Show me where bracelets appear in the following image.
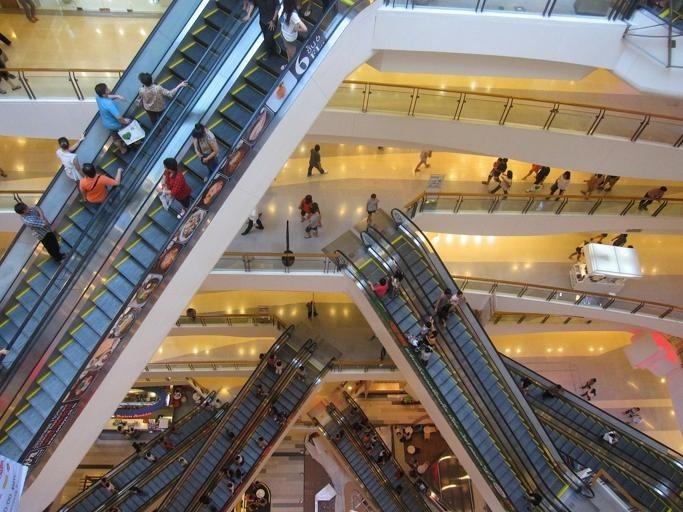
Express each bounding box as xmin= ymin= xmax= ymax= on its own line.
xmin=271 ymin=19 xmax=276 ymax=24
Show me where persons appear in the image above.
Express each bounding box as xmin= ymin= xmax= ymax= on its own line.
xmin=256 ymin=385 xmax=269 ymax=398
xmin=352 ymin=413 xmax=422 ymax=491
xmin=410 ymin=288 xmax=464 ymax=368
xmin=276 ymin=0 xmax=308 ymax=70
xmin=243 ymin=0 xmax=280 ymax=56
xmin=105 ymin=481 xmax=117 ymax=492
xmin=14 ymin=202 xmax=66 ymax=262
xmin=297 ymin=0 xmax=313 ymax=17
xmin=520 ymin=376 xmax=530 ymax=392
xmin=483 ymin=157 xmax=620 ymax=202
xmin=255 ymin=435 xmax=269 ymax=450
xmin=582 ymin=378 xmax=597 ymax=389
xmin=296 ymin=366 xmax=306 ymax=382
xmin=331 ymin=433 xmax=340 ymax=444
xmin=568 ymin=240 xmax=589 ymax=261
xmin=18 ymin=0 xmax=38 ymax=23
xmin=274 ymin=359 xmax=283 ymax=375
xmin=213 ymin=398 xmax=221 ymax=412
xmin=611 ymin=233 xmax=628 ymax=247
xmin=55 ymin=76 xmax=210 ymax=221
xmin=129 ymin=486 xmax=143 ymax=497
xmin=312 ymin=202 xmax=322 ymax=227
xmin=208 ymin=397 xmax=214 ymax=410
xmin=242 ymin=204 xmax=264 ymax=236
xmin=638 ymin=186 xmax=668 ymax=211
xmin=377 ymin=347 xmax=386 ymax=363
xmin=274 ymin=414 xmax=286 ymax=425
xmin=267 ymin=353 xmax=277 ymax=371
xmin=101 ymin=476 xmax=108 ymax=488
xmin=306 ymin=300 xmax=319 ymax=318
xmin=368 ymin=279 xmax=388 ymax=298
xmin=304 ymin=207 xmax=319 ymax=238
xmin=542 ymin=385 xmax=562 ymax=400
xmin=602 ymin=430 xmax=617 ymax=446
xmin=625 ymin=408 xmax=640 ymax=417
xmin=297 ymin=195 xmax=314 ymax=222
xmin=131 ymin=425 xmax=180 ymax=465
xmin=227 ymin=426 xmax=237 ymax=444
xmin=366 ymin=194 xmax=378 ymax=223
xmin=178 ymin=456 xmax=190 ymax=468
xmin=223 ymin=455 xmax=244 ymax=482
xmin=591 ymin=233 xmax=608 ymax=244
xmin=581 ymin=386 xmax=598 ymax=400
xmin=0 ymin=60 xmax=23 ymax=95
xmin=306 ymin=144 xmax=328 ymax=176
xmin=269 ymin=407 xmax=276 ymax=416
xmin=628 ymin=417 xmax=642 ymax=425
xmin=199 ymin=496 xmax=213 ymax=508
xmin=111 ymin=506 xmax=122 ymax=512
xmin=387 ymin=269 xmax=404 ymax=297
xmin=415 ymin=151 xmax=433 ymax=172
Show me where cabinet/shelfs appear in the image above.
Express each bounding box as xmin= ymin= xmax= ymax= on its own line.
xmin=569 ymin=242 xmax=642 ymax=298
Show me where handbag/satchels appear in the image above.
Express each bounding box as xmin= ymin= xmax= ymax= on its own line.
xmin=200 ymin=156 xmax=207 ymax=165
xmin=116 ymin=118 xmax=145 ymax=146
xmin=157 ymin=187 xmax=174 ymax=212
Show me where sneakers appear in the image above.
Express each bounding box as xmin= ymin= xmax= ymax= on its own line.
xmin=579 ymin=182 xmax=615 ymax=197
xmin=306 ymin=169 xmax=329 ymax=178
xmin=300 ymin=216 xmax=323 ymax=239
xmin=637 ymin=206 xmax=649 ymax=212
xmin=240 ymin=224 xmax=265 ymax=236
xmin=481 ymin=176 xmax=564 ymax=202
xmin=176 ymin=208 xmax=186 ymax=220
